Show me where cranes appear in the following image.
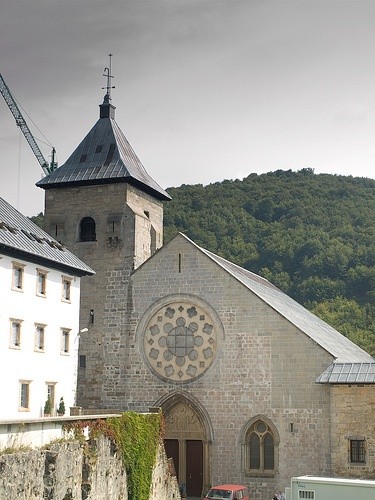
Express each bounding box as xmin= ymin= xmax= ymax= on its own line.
xmin=0 ymin=74 xmax=57 ymax=176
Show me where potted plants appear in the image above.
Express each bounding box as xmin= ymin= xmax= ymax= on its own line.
xmin=56 ymin=397 xmax=65 ymax=417
xmin=44 ymin=396 xmax=52 ymax=417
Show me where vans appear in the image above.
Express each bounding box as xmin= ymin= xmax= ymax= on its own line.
xmin=203 ymin=485 xmax=248 ymax=500
